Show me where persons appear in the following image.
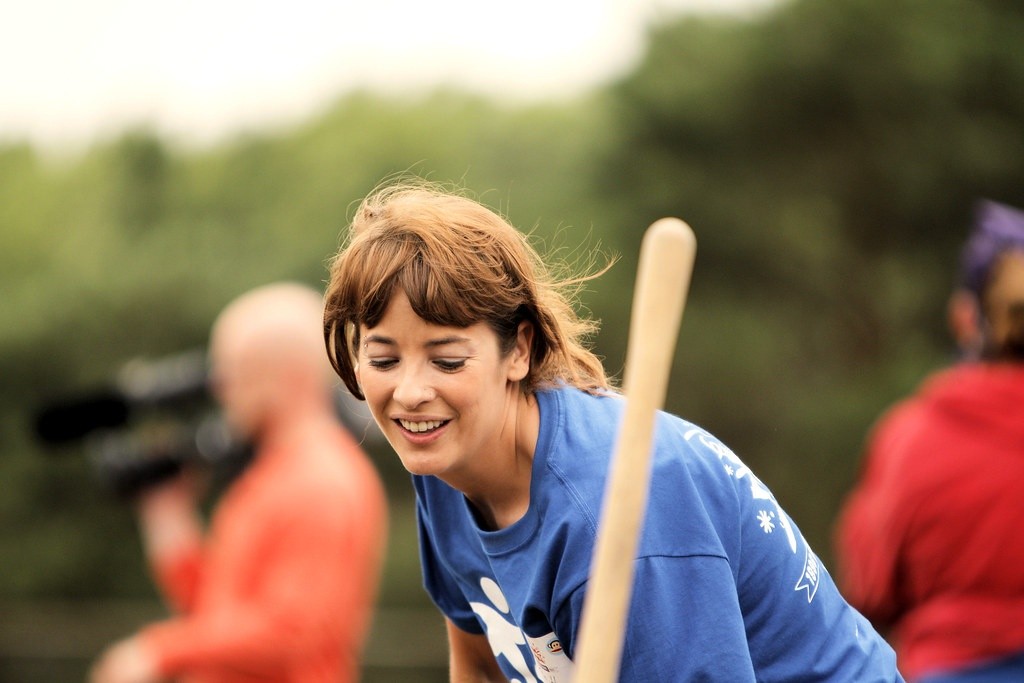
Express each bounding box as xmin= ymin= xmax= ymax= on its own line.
xmin=87 ymin=280 xmax=389 ymax=683
xmin=322 ymin=170 xmax=907 ymax=683
xmin=831 ymin=199 xmax=1024 ymax=683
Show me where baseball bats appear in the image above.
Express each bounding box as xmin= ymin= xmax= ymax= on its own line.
xmin=566 ymin=211 xmax=701 ymax=683
